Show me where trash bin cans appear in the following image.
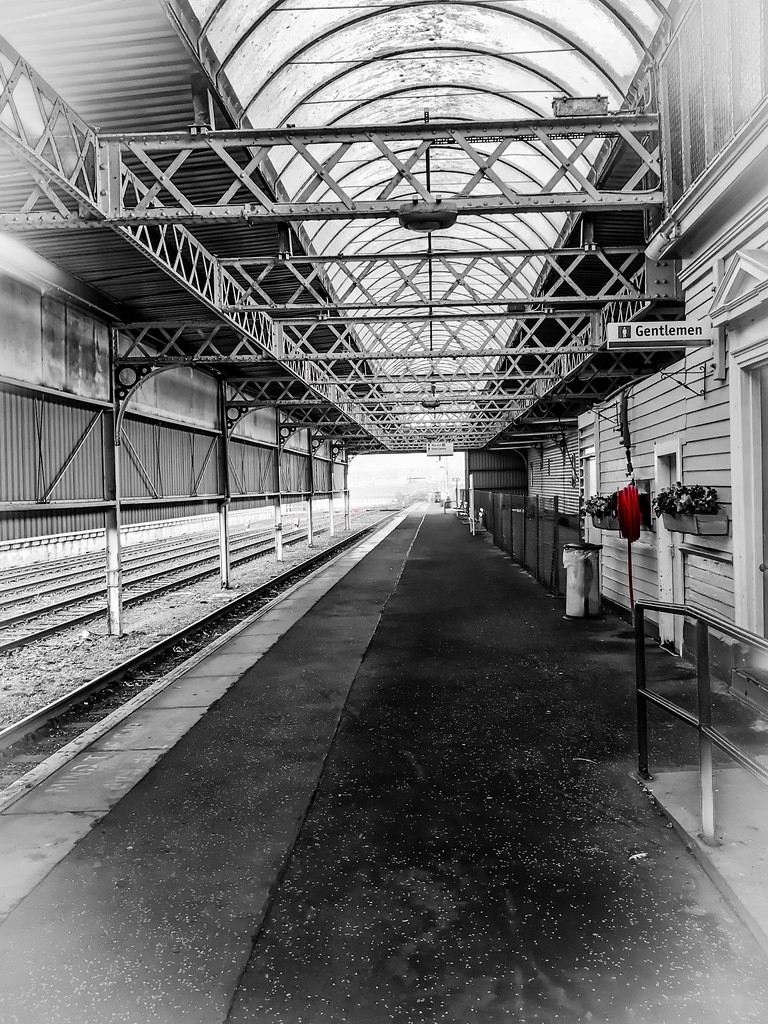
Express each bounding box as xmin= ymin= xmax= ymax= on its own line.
xmin=564 ymin=542 xmax=604 ymax=617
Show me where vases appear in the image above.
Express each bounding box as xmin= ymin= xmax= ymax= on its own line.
xmin=663 ymin=515 xmax=728 ymax=536
xmin=592 ymin=515 xmax=620 ymax=532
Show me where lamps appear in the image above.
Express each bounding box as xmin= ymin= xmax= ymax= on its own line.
xmin=644 ymin=226 xmax=677 ymax=261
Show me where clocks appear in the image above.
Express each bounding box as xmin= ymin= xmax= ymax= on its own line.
xmin=227 ymin=406 xmax=241 ymax=421
xmin=332 ymin=447 xmax=339 ymax=454
xmin=279 ymin=427 xmax=290 ymax=438
xmin=312 ymin=440 xmax=320 ymax=448
xmin=116 ymin=364 xmax=139 ymax=389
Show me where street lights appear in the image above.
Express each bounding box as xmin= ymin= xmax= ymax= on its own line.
xmin=440 ymin=465 xmax=449 ymax=494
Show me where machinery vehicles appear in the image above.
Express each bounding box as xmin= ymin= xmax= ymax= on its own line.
xmin=435 ymin=492 xmax=443 ymax=502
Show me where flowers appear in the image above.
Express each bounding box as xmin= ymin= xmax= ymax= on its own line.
xmin=648 ymin=483 xmax=723 ymax=520
xmin=586 ymin=490 xmax=621 ymax=518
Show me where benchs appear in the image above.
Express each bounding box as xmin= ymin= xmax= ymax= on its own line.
xmin=456 ymin=502 xmax=468 ymax=517
xmin=461 ymin=508 xmax=487 ymax=531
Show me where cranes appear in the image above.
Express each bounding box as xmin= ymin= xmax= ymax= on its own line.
xmin=406 ymin=476 xmax=426 ymax=485
